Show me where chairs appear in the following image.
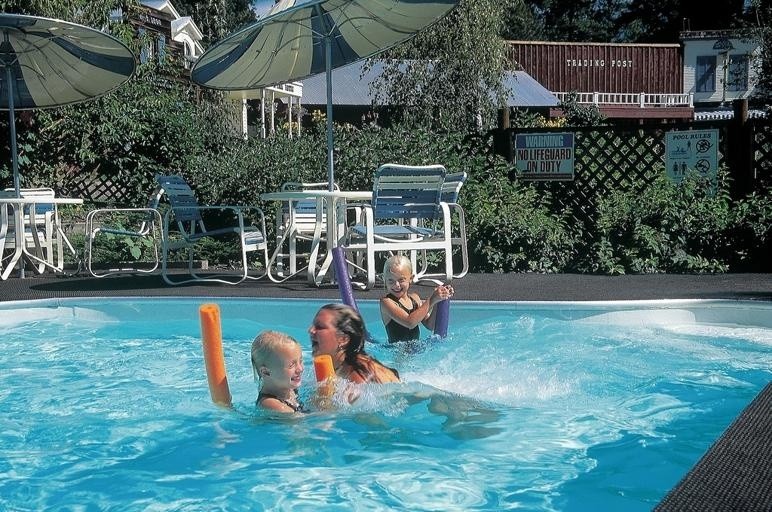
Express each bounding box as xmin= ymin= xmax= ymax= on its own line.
xmin=159 ymin=174 xmax=268 ymax=286
xmin=278 ymin=181 xmax=343 ymax=277
xmin=82 ymin=182 xmax=168 ymax=280
xmin=405 ymin=168 xmax=467 ymax=282
xmin=347 ymin=163 xmax=453 ymax=291
xmin=0 ymin=185 xmax=65 ymax=278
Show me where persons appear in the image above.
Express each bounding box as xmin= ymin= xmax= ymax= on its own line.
xmin=379 ymin=255 xmax=454 ymax=355
xmin=251 ymin=329 xmax=361 ymax=431
xmin=308 ymin=303 xmax=507 ymax=441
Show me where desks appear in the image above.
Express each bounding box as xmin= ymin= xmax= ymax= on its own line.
xmin=262 ymin=187 xmax=403 ymax=287
xmin=0 ymin=197 xmax=88 ymax=281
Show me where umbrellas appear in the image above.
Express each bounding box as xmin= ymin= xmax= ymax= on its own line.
xmin=0 ymin=13 xmax=137 ymax=197
xmin=190 ymin=0 xmax=461 ymax=191
